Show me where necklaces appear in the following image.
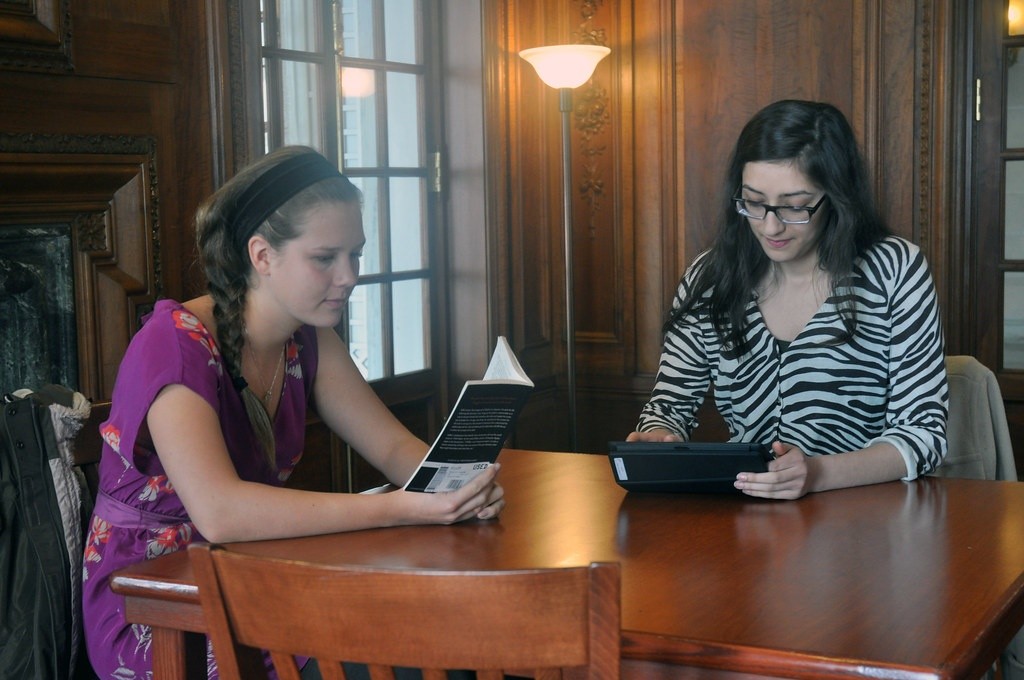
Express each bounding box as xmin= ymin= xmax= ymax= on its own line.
xmin=237 ymin=316 xmax=289 ymax=410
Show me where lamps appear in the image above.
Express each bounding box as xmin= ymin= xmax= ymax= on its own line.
xmin=519 ymin=43 xmax=613 ymax=450
xmin=1008 ymin=0 xmax=1024 ymax=67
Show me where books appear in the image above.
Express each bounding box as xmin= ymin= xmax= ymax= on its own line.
xmin=401 ymin=337 xmax=537 ymax=496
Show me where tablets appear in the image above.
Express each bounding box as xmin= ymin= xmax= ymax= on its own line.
xmin=608 ymin=440 xmax=776 ymax=495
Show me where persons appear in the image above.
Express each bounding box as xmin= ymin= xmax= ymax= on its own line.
xmin=82 ymin=145 xmax=508 ymax=680
xmin=627 ymin=98 xmax=948 ymax=502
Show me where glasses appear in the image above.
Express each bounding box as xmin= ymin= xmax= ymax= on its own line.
xmin=731 ymin=182 xmax=828 ymax=224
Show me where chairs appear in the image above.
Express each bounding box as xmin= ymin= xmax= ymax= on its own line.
xmin=0 ymin=398 xmax=112 ymax=680
xmin=185 ymin=543 xmax=623 ymax=679
xmin=924 ymin=356 xmax=1017 ymax=482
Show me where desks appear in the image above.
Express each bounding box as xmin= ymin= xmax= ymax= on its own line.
xmin=112 ymin=450 xmax=1024 ymax=680
